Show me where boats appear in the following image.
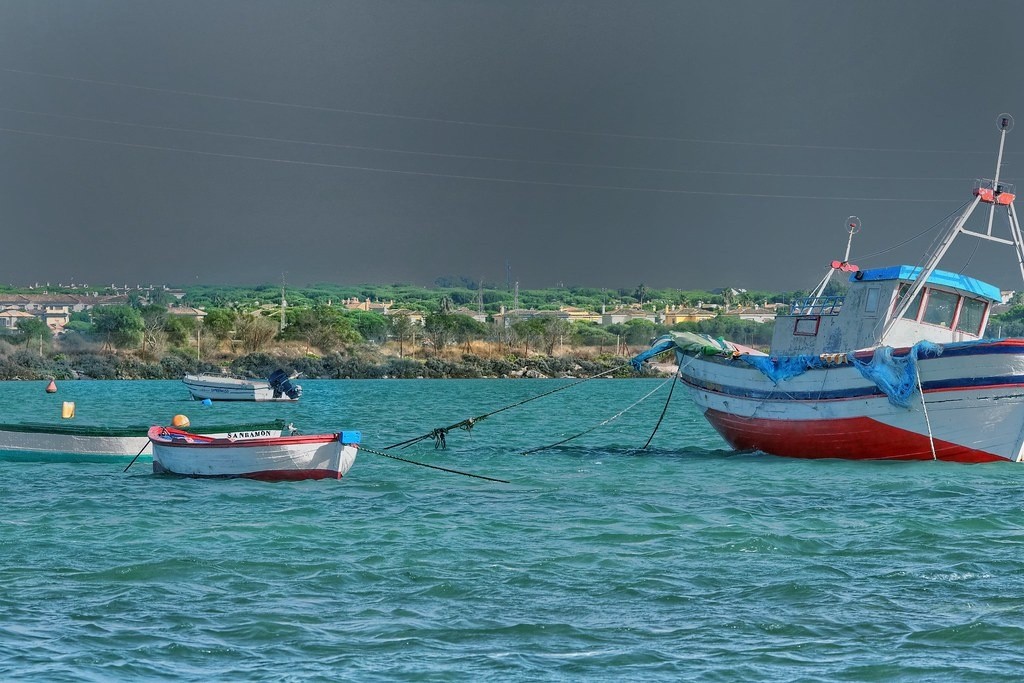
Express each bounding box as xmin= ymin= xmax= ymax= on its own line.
xmin=0 ymin=419 xmax=186 ymax=462
xmin=182 ymin=368 xmax=304 ymax=402
xmin=669 ymin=112 xmax=1024 ymax=462
xmin=148 ymin=424 xmax=360 ymax=483
